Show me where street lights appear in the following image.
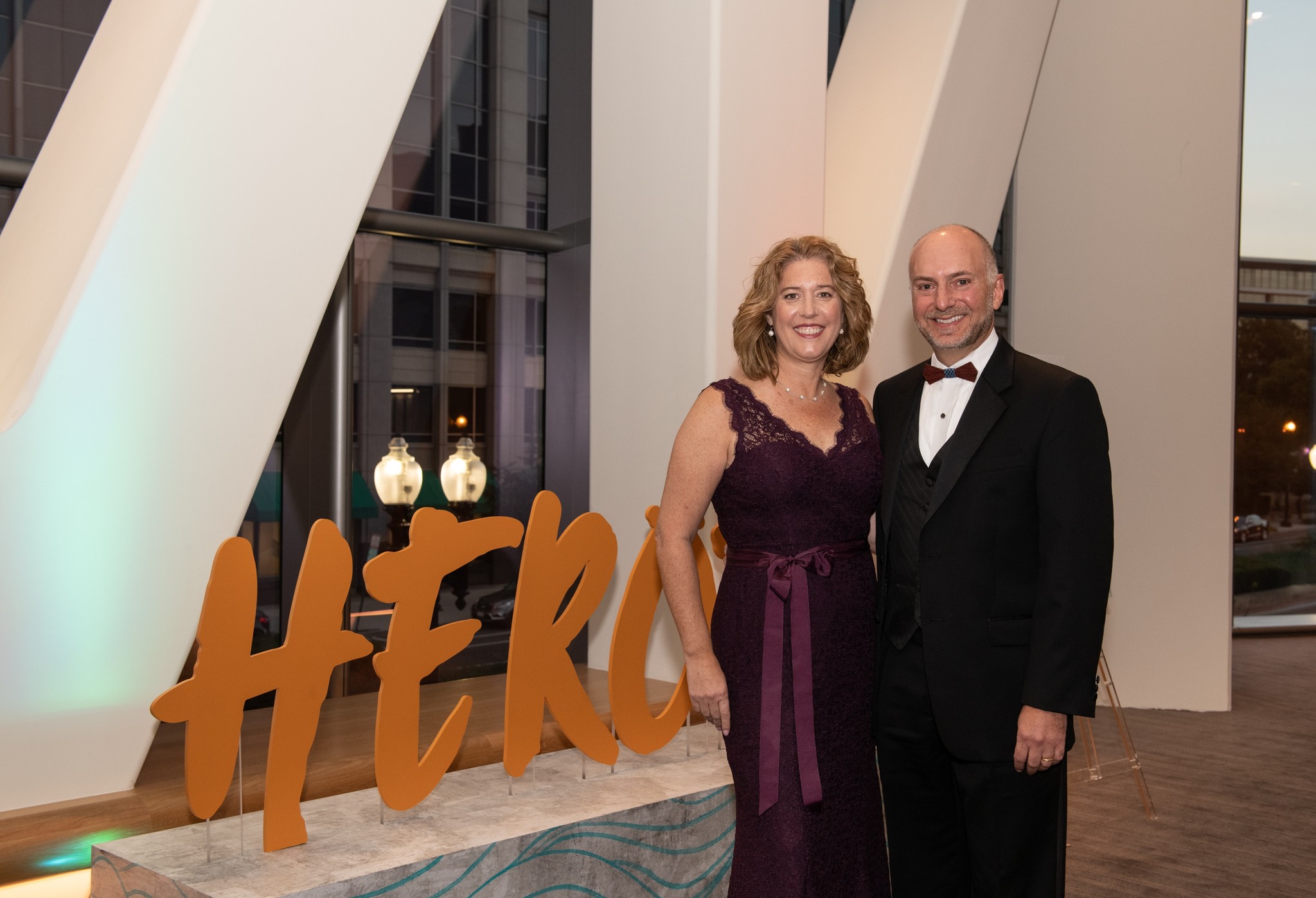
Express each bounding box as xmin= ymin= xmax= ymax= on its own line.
xmin=374 ymin=437 xmax=487 ymax=686
xmin=1280 ymin=420 xmax=1296 ymax=527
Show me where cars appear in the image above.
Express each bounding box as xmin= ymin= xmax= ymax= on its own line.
xmin=1233 ymin=514 xmax=1268 ymax=543
xmin=250 ymin=607 xmax=273 ymax=656
xmin=470 ymin=582 xmax=518 ymax=629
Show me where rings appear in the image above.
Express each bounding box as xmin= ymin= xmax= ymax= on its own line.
xmin=1041 ymin=758 xmax=1054 ymax=761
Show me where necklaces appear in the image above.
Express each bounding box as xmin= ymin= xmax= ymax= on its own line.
xmin=773 ymin=370 xmax=826 ymax=402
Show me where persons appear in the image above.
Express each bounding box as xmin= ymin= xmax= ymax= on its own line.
xmin=656 ymin=236 xmax=876 ymax=898
xmin=872 ymin=222 xmax=1115 ymax=898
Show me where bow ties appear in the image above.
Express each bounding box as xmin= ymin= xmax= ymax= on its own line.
xmin=923 ymin=361 xmax=978 ymax=385
